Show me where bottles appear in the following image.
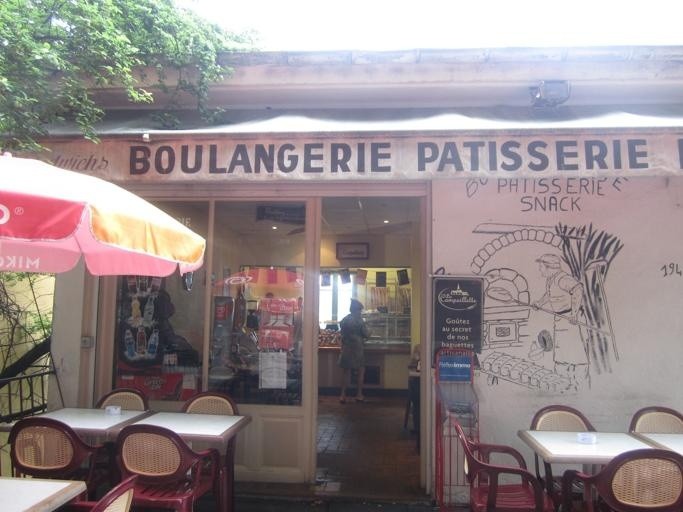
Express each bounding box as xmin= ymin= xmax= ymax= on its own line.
xmin=130 ymin=296 xmax=140 ymax=326
xmin=146 ymin=329 xmax=161 ymax=358
xmin=148 ymin=277 xmax=161 ymax=297
xmin=135 ymin=328 xmax=146 ymax=357
xmin=124 ymin=326 xmax=136 ymax=358
xmin=142 ymin=297 xmax=154 ymax=327
xmin=138 ymin=276 xmax=147 ymax=296
xmin=126 ymin=276 xmax=137 ymax=294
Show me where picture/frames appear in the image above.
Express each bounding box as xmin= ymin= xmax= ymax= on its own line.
xmin=336 ymin=242 xmax=369 ymax=260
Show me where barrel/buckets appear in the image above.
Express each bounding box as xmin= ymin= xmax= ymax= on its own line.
xmin=214 ymin=295 xmax=234 ymax=338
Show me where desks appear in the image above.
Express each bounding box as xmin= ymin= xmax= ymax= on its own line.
xmin=0 ymin=475 xmax=86 ymax=512
xmin=409 ymin=372 xmax=420 ymax=454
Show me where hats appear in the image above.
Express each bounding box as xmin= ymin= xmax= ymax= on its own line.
xmin=349 ymin=299 xmax=363 ymax=312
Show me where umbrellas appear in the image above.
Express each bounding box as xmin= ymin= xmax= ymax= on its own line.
xmin=0 ymin=145 xmax=208 ymax=294
xmin=212 ymin=263 xmax=306 ymax=298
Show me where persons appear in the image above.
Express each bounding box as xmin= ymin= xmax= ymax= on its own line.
xmin=336 ymin=297 xmax=378 ymax=402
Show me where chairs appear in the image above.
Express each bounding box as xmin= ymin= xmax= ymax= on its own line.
xmin=531 ymin=405 xmax=597 ymax=512
xmin=455 ymin=422 xmax=556 ymax=512
xmin=179 ymin=392 xmax=239 ymax=512
xmin=115 ymin=424 xmax=221 ymax=512
xmin=562 ymin=448 xmax=683 ymax=512
xmin=66 ymin=474 xmax=140 ymax=512
xmin=9 ymin=417 xmax=112 ymax=501
xmin=629 ymin=406 xmax=683 ymax=433
xmin=89 ymin=387 xmax=148 ymax=490
